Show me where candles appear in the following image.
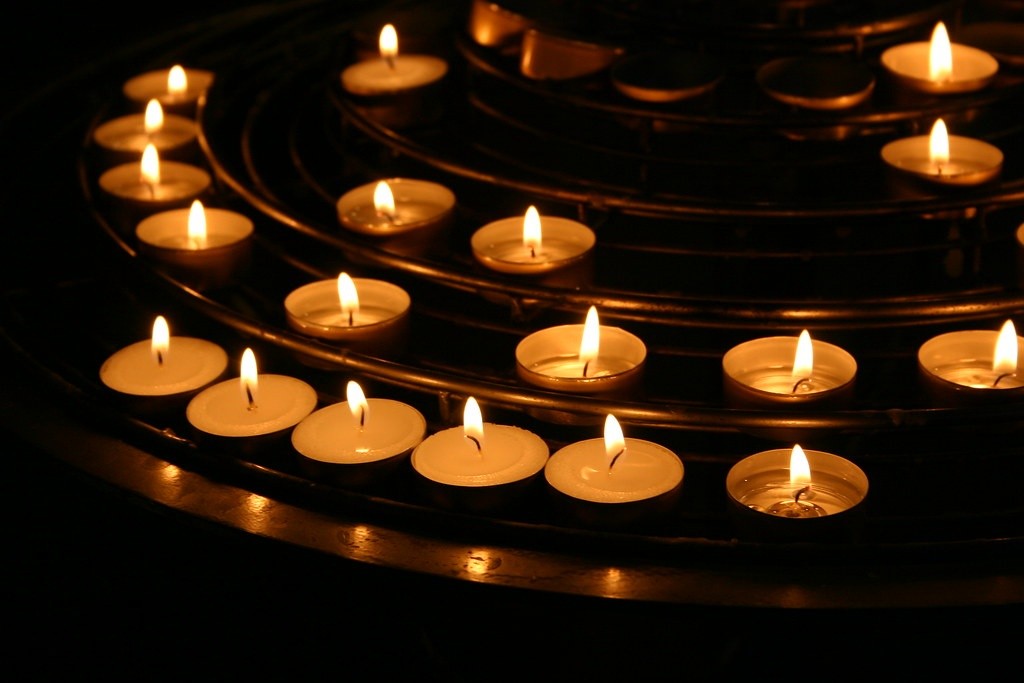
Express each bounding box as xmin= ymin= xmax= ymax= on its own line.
xmin=98 ymin=314 xmax=230 ymax=435
xmin=335 ymin=176 xmax=458 ymax=262
xmin=514 ymin=304 xmax=651 ymax=397
xmin=914 ymin=317 xmax=1024 ymax=411
xmin=879 ymin=21 xmax=999 ymax=109
xmin=407 ymin=397 xmax=553 ymax=516
xmin=721 ymin=327 xmax=861 ymax=412
xmin=97 ymin=143 xmax=213 ymax=245
xmin=90 ymin=98 xmax=201 ymax=183
xmin=542 ymin=414 xmax=687 ymax=536
xmin=468 ymin=204 xmax=595 ymax=292
xmin=338 ymin=21 xmax=453 ymax=133
xmin=726 ymin=444 xmax=868 ymax=542
xmin=284 ymin=271 xmax=413 ymax=362
xmin=184 ymin=347 xmax=320 ymax=469
xmin=122 ymin=62 xmax=216 ymax=121
xmin=879 ymin=115 xmax=1005 ymax=202
xmin=135 ymin=199 xmax=256 ymax=301
xmin=288 ymin=377 xmax=426 ymax=497
